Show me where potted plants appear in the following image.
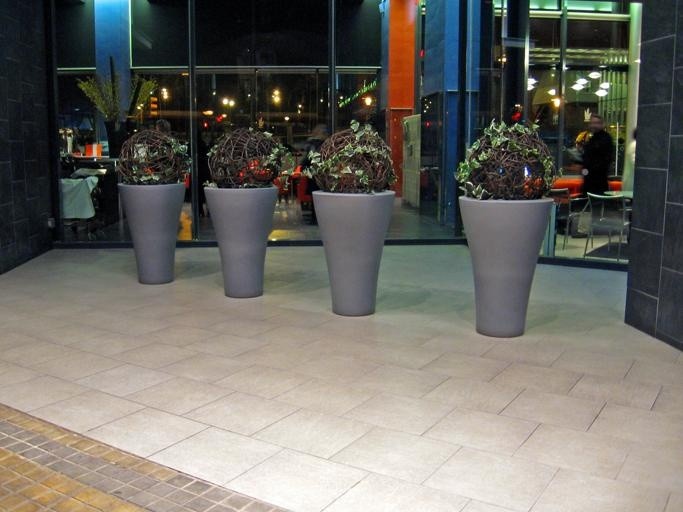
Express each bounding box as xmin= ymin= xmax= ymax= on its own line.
xmin=204 ymin=128 xmax=285 ymax=299
xmin=115 ymin=120 xmax=190 ymax=284
xmin=304 ymin=121 xmax=400 ymax=315
xmin=451 ymin=116 xmax=558 ymax=340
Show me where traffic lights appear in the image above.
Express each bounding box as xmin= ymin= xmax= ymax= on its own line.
xmin=146 ymin=97 xmax=158 ymax=118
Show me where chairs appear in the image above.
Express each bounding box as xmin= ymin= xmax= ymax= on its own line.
xmin=548 ymin=189 xmax=631 ymax=265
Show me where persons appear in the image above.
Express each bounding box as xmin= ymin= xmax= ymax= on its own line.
xmin=575 ymin=113 xmax=614 ymax=196
xmin=155 ymin=118 xmax=171 ymax=133
xmin=300 ymin=123 xmax=329 ymax=171
xmin=621 ymin=128 xmax=637 ymax=243
